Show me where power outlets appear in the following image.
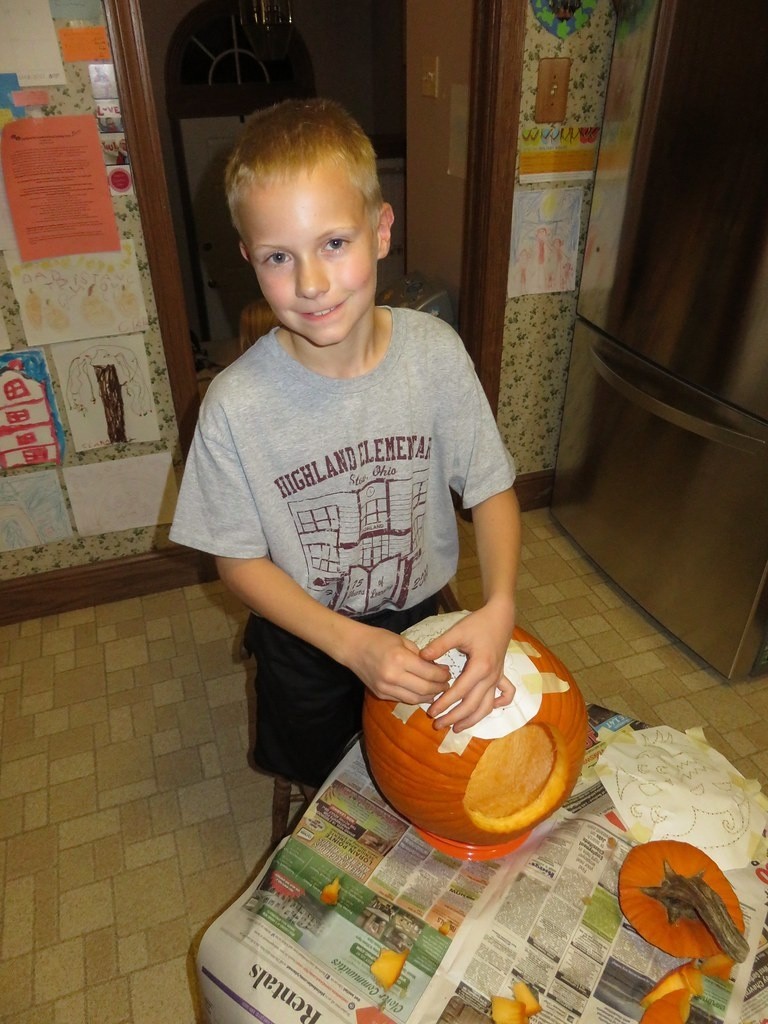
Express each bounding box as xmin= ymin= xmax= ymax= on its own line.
xmin=534 ymin=58 xmax=571 ymax=123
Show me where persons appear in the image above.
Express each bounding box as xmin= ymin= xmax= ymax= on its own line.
xmin=168 ymin=98 xmax=520 ymax=790
xmin=238 ymin=298 xmax=282 ymax=353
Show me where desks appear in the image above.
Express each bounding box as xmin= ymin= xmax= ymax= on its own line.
xmin=196 ymin=703 xmax=768 ymax=1024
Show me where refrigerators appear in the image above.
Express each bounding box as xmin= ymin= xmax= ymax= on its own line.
xmin=550 ymin=0 xmax=768 ymax=683
